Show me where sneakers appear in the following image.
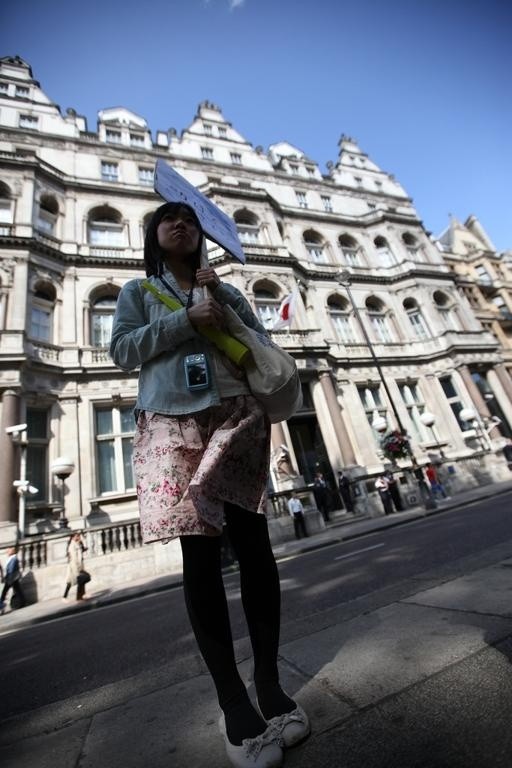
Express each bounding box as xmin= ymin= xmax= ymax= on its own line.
xmin=217 ymin=709 xmax=282 ymax=768
xmin=256 ymin=688 xmax=310 ymax=746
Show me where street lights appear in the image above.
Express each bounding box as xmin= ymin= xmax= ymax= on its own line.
xmin=419 ymin=410 xmax=454 ymax=465
xmin=50 ymin=456 xmax=77 ymax=535
xmin=333 ymin=266 xmax=437 ymax=512
xmin=458 ymin=405 xmax=492 ymax=459
xmin=370 ymin=407 xmax=404 ymax=472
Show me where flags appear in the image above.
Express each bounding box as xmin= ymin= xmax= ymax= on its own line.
xmin=271 ymin=291 xmax=297 ymax=331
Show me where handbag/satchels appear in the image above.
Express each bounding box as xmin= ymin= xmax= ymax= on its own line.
xmin=222 ymin=300 xmax=304 ymax=424
xmin=77 ymin=570 xmax=90 ymax=584
xmin=5 ymin=570 xmax=21 ymax=585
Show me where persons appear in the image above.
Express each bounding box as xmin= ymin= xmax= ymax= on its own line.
xmin=387 ymin=473 xmax=403 ymax=511
xmin=0 ymin=548 xmax=24 ymax=615
xmin=337 ymin=470 xmax=353 ymax=514
xmin=287 ymin=491 xmax=308 ymax=539
xmin=313 ymin=471 xmax=330 ymax=522
xmin=63 ymin=533 xmax=90 ymax=604
xmin=106 ymin=201 xmax=310 ymax=766
xmin=425 ymin=462 xmax=451 ymax=502
xmin=374 ymin=473 xmax=394 ymax=517
xmin=435 ymin=463 xmax=455 ymax=500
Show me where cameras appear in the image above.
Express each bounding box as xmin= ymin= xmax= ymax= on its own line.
xmin=184 ymin=353 xmax=210 ymax=391
